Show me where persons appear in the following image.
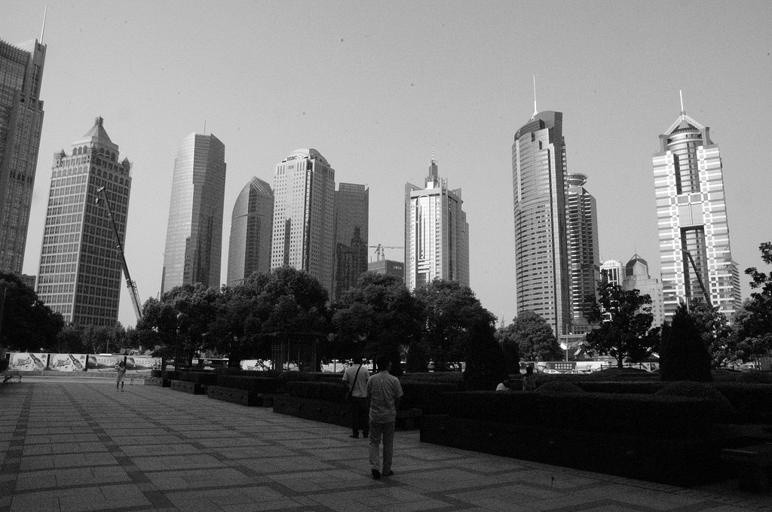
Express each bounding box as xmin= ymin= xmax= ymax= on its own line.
xmin=115 ymin=358 xmax=126 ymax=392
xmin=341 ymin=354 xmax=370 ymax=440
xmin=495 ymin=375 xmax=512 ymax=393
xmin=365 ymin=354 xmax=406 ymax=482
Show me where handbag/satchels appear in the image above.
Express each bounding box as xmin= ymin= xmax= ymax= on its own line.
xmin=344 ymin=392 xmax=352 ymax=403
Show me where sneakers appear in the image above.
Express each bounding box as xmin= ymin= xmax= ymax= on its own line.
xmin=348 ymin=434 xmax=397 ymax=481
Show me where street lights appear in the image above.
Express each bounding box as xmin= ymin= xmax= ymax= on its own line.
xmin=566 ymin=323 xmax=573 ymax=363
